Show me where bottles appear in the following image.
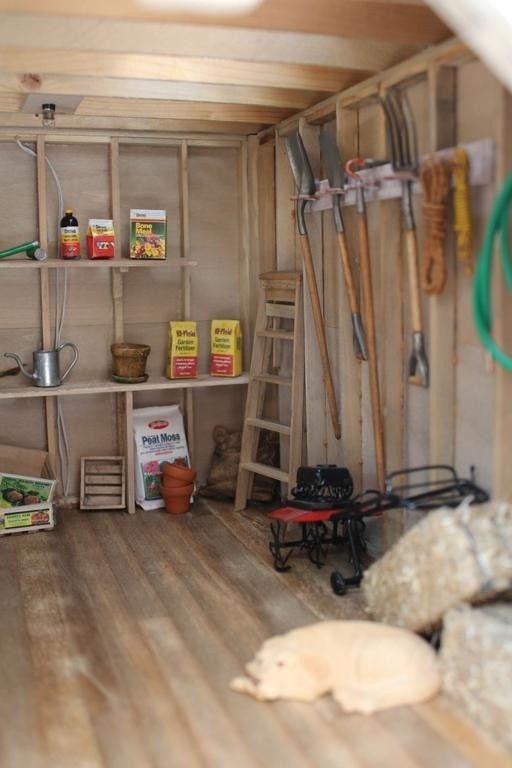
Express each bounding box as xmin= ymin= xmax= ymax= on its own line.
xmin=59 ymin=208 xmax=81 ymax=260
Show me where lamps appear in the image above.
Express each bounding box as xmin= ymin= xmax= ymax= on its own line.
xmin=24 ymin=92 xmax=81 ymax=128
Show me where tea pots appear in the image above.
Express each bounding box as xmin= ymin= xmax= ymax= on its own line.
xmin=2 ymin=342 xmax=78 ymax=390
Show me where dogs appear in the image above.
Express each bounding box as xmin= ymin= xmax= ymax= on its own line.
xmin=229 ymin=619 xmax=444 ymax=717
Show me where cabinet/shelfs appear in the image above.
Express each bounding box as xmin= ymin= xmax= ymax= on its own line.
xmin=0 ymin=259 xmax=253 ymax=400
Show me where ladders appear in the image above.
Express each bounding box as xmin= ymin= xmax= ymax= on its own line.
xmin=235 ymin=272 xmax=303 ymax=511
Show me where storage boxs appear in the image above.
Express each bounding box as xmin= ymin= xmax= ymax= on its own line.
xmin=0 ymin=446 xmax=57 ymax=535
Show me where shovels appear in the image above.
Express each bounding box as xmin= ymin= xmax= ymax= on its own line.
xmin=283 ymin=132 xmax=342 ymax=440
xmin=317 ymin=131 xmax=369 ymax=361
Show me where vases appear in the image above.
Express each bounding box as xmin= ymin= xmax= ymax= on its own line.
xmin=161 ymin=460 xmax=196 ymax=486
xmin=111 ymin=341 xmax=150 ymax=382
xmin=159 ymin=484 xmax=194 ymax=514
